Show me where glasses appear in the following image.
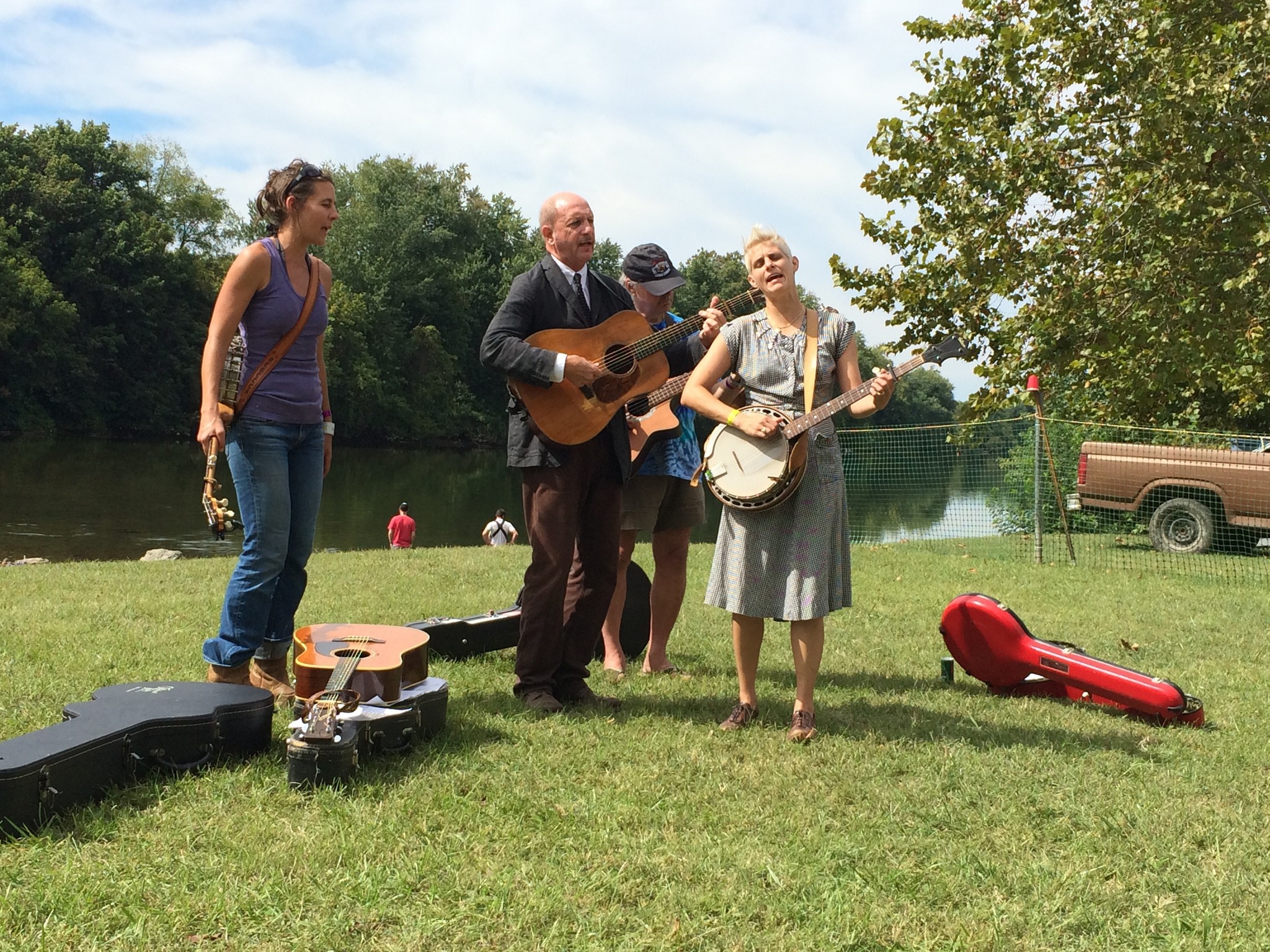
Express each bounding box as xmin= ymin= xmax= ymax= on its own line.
xmin=282 ymin=164 xmax=323 ymax=202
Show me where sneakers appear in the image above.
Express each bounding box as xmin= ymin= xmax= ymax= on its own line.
xmin=786 ymin=709 xmax=816 ymax=746
xmin=719 ymin=702 xmax=760 ymax=730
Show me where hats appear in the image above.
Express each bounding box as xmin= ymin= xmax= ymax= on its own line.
xmin=621 ymin=243 xmax=687 ymax=296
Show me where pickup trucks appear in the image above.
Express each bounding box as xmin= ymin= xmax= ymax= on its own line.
xmin=1064 ymin=442 xmax=1269 ymax=556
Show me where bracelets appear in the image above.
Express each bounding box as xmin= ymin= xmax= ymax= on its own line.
xmin=726 ymin=374 xmax=743 ymax=391
xmin=322 ymin=411 xmax=331 ymax=417
xmin=728 ymin=410 xmax=741 ymax=426
xmin=323 ymin=421 xmax=335 ymax=435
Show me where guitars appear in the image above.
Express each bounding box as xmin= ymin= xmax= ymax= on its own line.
xmin=509 ymin=287 xmax=765 ymax=448
xmin=703 ymin=333 xmax=972 ymax=512
xmin=622 ymin=370 xmax=695 ymax=480
xmin=291 ymin=624 xmax=430 ymax=742
xmin=195 ymin=335 xmax=248 ymax=542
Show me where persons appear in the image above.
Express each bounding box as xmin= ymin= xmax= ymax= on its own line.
xmin=482 ymin=508 xmax=518 ymax=545
xmin=601 ymin=244 xmax=746 ymax=682
xmin=196 ymin=159 xmax=341 ymax=705
xmin=483 ymin=194 xmax=727 ymax=714
xmin=682 ymin=223 xmax=897 ymax=743
xmin=388 ymin=503 xmax=416 ymax=550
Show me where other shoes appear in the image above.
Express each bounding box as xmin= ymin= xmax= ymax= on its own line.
xmin=555 ymin=678 xmax=621 ymax=708
xmin=514 ymin=676 xmax=564 ymax=713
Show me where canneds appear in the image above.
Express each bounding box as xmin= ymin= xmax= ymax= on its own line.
xmin=941 ymin=657 xmax=954 ymax=683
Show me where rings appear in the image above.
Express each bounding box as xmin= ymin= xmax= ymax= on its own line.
xmin=761 ymin=427 xmax=766 ymax=433
xmin=877 ymin=391 xmax=885 ymax=396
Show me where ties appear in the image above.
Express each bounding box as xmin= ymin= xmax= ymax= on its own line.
xmin=573 ymin=273 xmax=592 ymax=328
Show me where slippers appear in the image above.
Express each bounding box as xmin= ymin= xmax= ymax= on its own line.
xmin=641 ymin=664 xmax=692 ymax=681
xmin=602 ymin=668 xmax=627 ymax=682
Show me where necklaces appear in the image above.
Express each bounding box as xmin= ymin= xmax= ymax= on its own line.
xmin=764 ymin=303 xmax=804 ymax=329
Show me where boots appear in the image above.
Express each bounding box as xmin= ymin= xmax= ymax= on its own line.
xmin=208 ymin=660 xmax=254 ymax=687
xmin=250 ymin=655 xmax=296 ymax=705
